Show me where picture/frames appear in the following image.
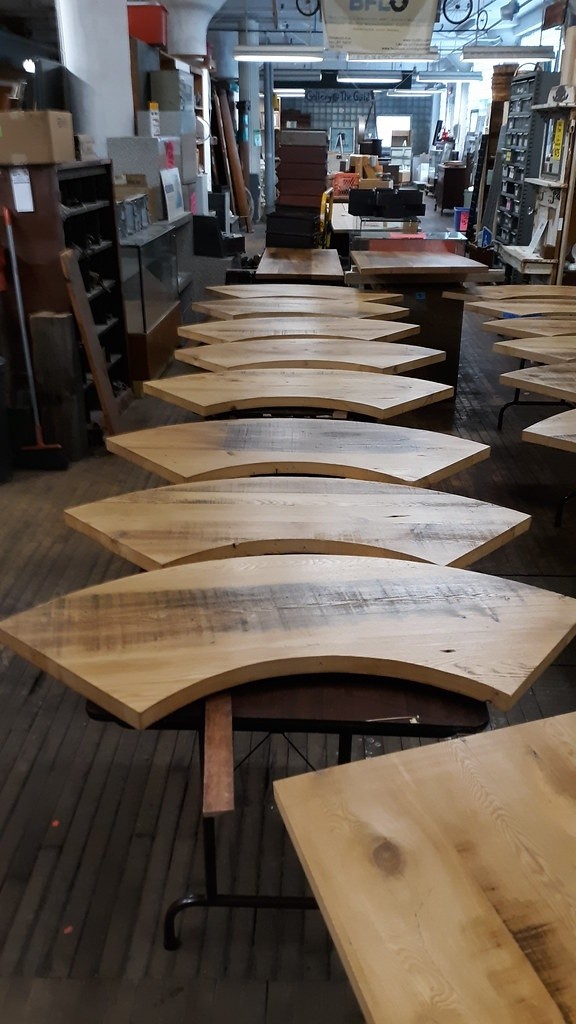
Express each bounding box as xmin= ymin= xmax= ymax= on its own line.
xmin=328 ymin=127 xmax=355 ymax=154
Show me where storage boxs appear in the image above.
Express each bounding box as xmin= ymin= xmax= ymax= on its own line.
xmin=116 ymin=187 xmax=160 ymax=223
xmin=454 ymin=206 xmax=469 ymax=232
xmin=0 ymin=110 xmax=75 ymax=166
xmin=127 ymin=1 xmax=170 ymax=47
xmin=116 ymin=196 xmax=150 ymax=236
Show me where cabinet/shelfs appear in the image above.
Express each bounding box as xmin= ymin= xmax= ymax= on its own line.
xmin=497 ymin=101 xmax=576 ymax=286
xmin=0 ymin=159 xmax=134 ymax=463
xmin=120 ymin=226 xmax=181 ymax=381
xmin=434 ymin=165 xmax=466 ymax=214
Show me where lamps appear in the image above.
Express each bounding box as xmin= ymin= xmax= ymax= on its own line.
xmin=461 ymin=0 xmax=555 ymax=62
xmin=231 ymin=0 xmax=327 ymax=62
xmin=260 ymin=64 xmax=482 ymax=98
xmin=345 ymin=45 xmax=441 ymax=63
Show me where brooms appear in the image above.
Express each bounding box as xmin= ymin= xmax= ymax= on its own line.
xmin=0 ymin=205 xmax=70 ymax=473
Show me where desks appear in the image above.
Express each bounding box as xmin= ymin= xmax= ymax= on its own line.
xmin=201 ymin=284 xmax=403 ymax=303
xmin=462 ymin=301 xmax=576 ymax=319
xmin=522 ymin=409 xmax=576 ymax=453
xmin=350 ymin=249 xmax=490 ymax=275
xmin=190 ymin=298 xmax=411 ymax=321
xmin=326 ymin=195 xmax=388 ymax=228
xmin=169 ymin=340 xmax=445 ymax=373
xmin=357 ymin=216 xmax=421 ymax=232
xmin=65 ymin=475 xmax=533 ymax=572
xmin=273 ymin=709 xmax=576 ymax=1024
xmin=478 ymin=319 xmax=576 ymax=340
xmin=103 ymin=416 xmax=489 ymax=487
xmin=492 ymin=336 xmax=576 ymax=431
xmin=1 ymin=551 xmax=576 ymax=950
xmin=140 ymin=366 xmax=453 ymax=422
xmin=176 ymin=317 xmax=420 ymax=342
xmin=254 ymin=248 xmax=344 ymax=281
xmin=499 ymin=363 xmax=576 ymax=402
xmin=442 ymin=280 xmax=576 ymax=301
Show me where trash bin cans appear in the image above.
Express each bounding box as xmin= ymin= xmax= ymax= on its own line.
xmin=453 ymin=207 xmax=478 ymax=232
